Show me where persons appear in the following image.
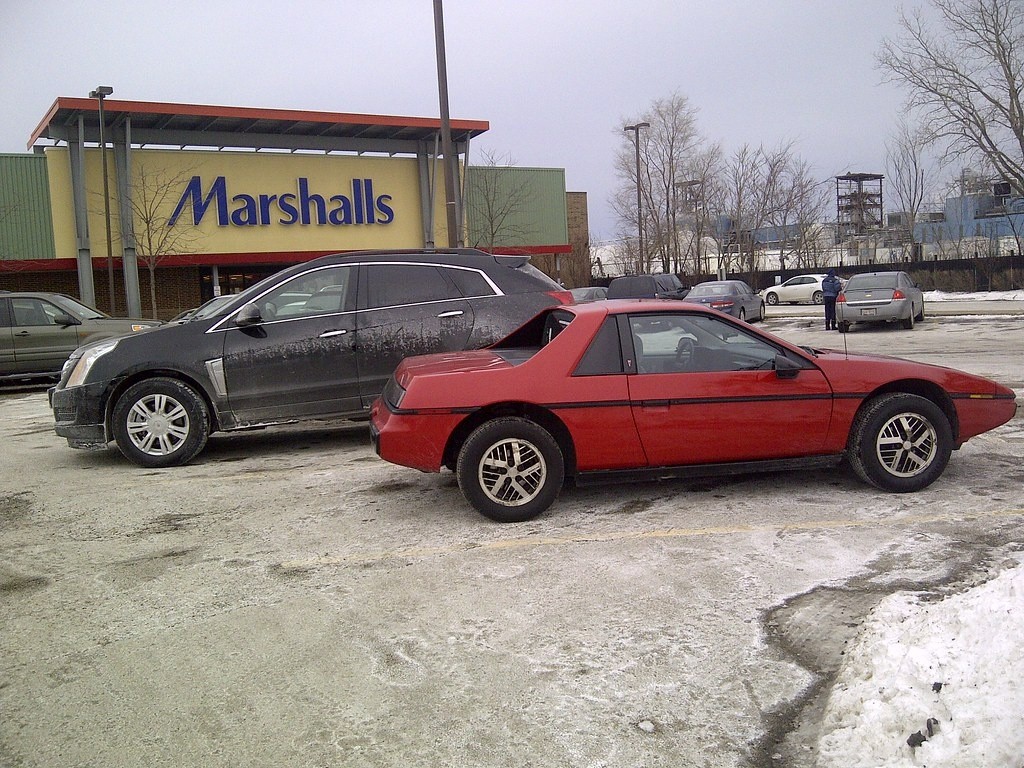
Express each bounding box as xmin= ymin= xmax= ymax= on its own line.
xmin=822 ymin=268 xmax=843 ymax=330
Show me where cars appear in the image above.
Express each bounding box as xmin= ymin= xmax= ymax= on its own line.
xmin=763 ymin=272 xmax=848 ymax=304
xmin=559 ymin=272 xmax=767 ymax=363
xmin=45 ymin=245 xmax=578 ymax=467
xmin=834 ymin=269 xmax=926 ymax=333
xmin=367 ymin=295 xmax=1017 ymax=523
xmin=0 ymin=289 xmax=169 ymax=393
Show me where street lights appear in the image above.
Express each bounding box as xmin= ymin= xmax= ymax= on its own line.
xmin=623 ymin=122 xmax=650 ymax=274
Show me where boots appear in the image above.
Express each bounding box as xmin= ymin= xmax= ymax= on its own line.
xmin=831 ymin=321 xmax=838 ymax=330
xmin=825 ymin=320 xmax=830 ymax=331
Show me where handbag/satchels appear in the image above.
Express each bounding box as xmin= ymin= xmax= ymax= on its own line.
xmin=837 ymin=289 xmax=846 ymax=302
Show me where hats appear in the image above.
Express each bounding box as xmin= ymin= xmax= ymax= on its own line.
xmin=825 ymin=268 xmax=835 ymax=276
